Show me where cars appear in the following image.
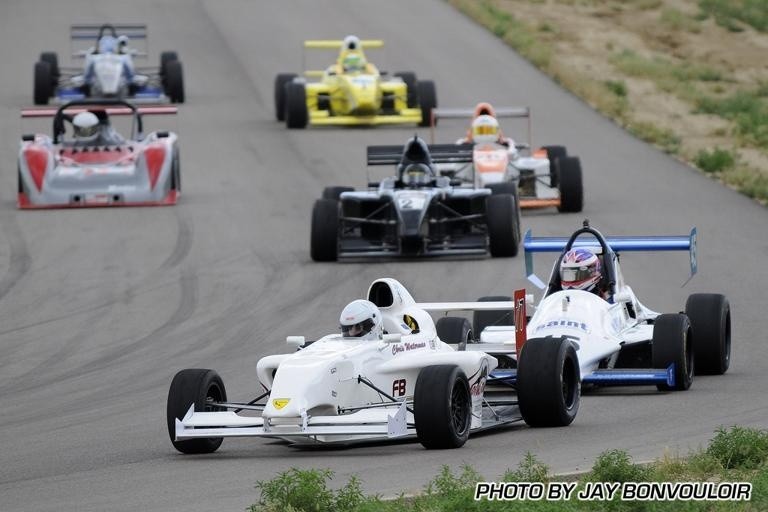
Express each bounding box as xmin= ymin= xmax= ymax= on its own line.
xmin=19 ymin=21 xmax=184 ymax=119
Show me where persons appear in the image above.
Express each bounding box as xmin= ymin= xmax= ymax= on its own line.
xmin=336 ymin=298 xmax=385 ymax=342
xmin=95 ymin=34 xmax=120 ymax=55
xmin=399 ymin=162 xmax=433 ymax=186
xmin=338 ymin=50 xmax=368 ymax=74
xmin=466 ymin=113 xmax=504 ymax=146
xmin=555 ymin=248 xmax=605 ymax=294
xmin=71 ymin=111 xmax=102 ymax=142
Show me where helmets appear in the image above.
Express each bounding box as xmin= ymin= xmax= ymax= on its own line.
xmin=559 ymin=247 xmax=603 ymax=292
xmin=98 ymin=35 xmax=118 ymax=54
xmin=72 ymin=112 xmax=100 ymax=142
xmin=340 ymin=299 xmax=384 ymax=341
xmin=472 ymin=115 xmax=500 ymax=144
xmin=402 ymin=162 xmax=433 ymax=187
